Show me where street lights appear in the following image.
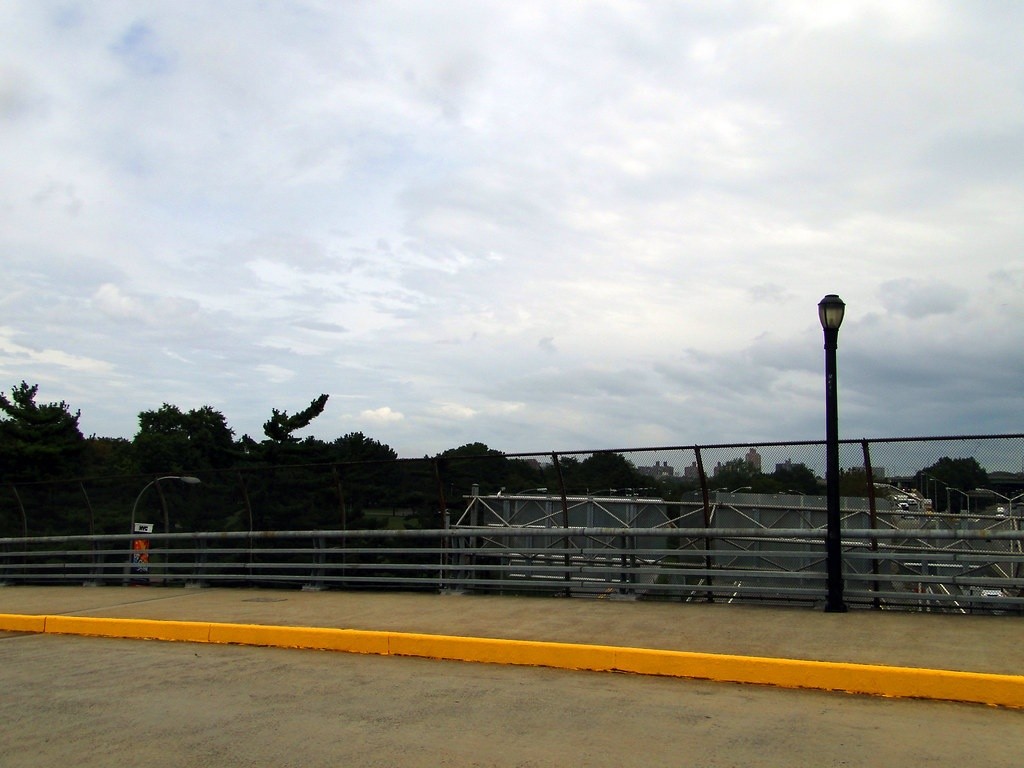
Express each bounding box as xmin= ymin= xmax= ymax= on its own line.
xmin=945 ymin=487 xmax=990 ymax=530
xmin=514 ymin=487 xmax=549 ymax=529
xmin=129 ymin=476 xmax=201 ymax=561
xmin=817 ymin=293 xmax=847 ymax=613
xmin=976 ymin=488 xmax=1024 ymax=577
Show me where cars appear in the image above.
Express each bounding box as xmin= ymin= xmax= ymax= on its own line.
xmin=996 ymin=504 xmax=1017 ymax=520
